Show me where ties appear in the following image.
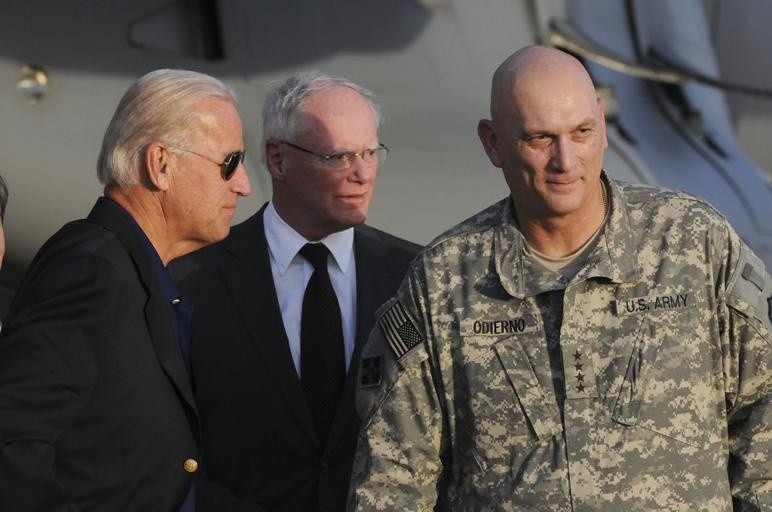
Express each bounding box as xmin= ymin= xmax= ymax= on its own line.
xmin=297 ymin=240 xmax=347 ymax=447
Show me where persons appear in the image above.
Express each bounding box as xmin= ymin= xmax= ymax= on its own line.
xmin=347 ymin=46 xmax=771 ymax=512
xmin=1 ymin=63 xmax=249 ymax=512
xmin=165 ymin=68 xmax=426 ymax=512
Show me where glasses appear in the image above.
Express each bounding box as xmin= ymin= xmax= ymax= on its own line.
xmin=164 ymin=143 xmax=246 ymax=182
xmin=277 ymin=140 xmax=390 ymax=172
